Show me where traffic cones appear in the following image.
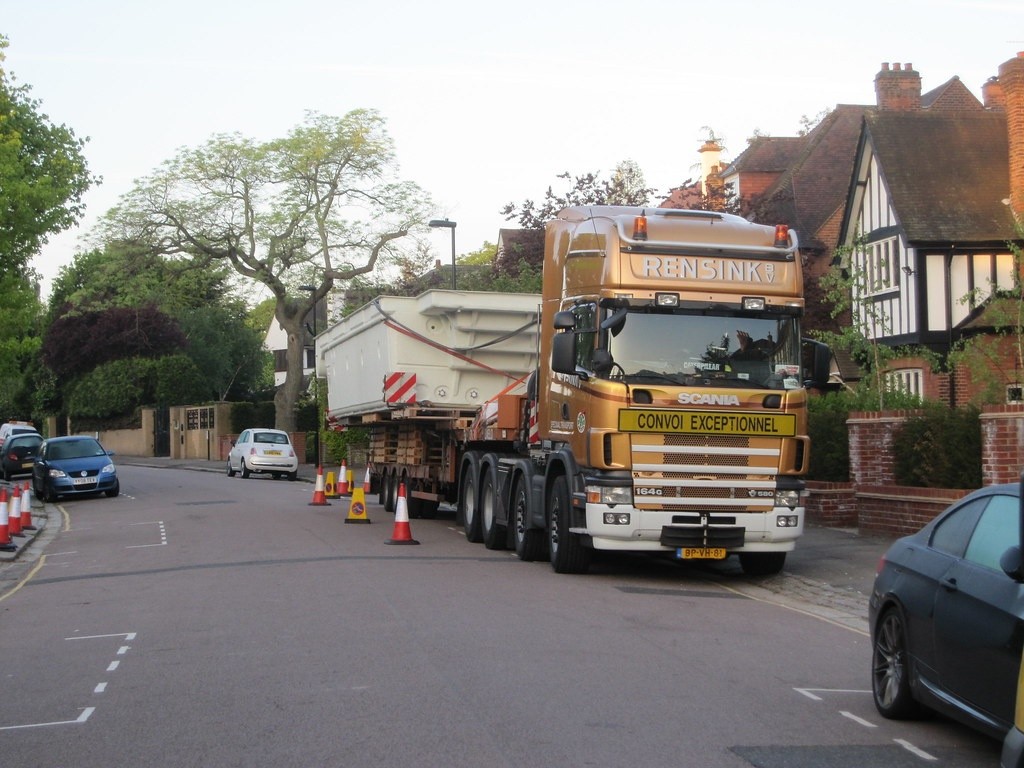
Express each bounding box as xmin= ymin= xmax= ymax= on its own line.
xmin=384 ymin=481 xmax=421 ymax=546
xmin=308 ymin=464 xmax=331 ymax=506
xmin=0 ymin=488 xmax=18 ymax=549
xmin=363 ymin=463 xmax=377 ymax=495
xmin=336 ymin=458 xmax=351 ymax=497
xmin=20 ymin=481 xmax=37 ymax=530
xmin=8 ymin=483 xmax=27 ymax=537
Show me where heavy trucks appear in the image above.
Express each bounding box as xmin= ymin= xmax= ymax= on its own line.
xmin=312 ymin=206 xmax=810 ymax=576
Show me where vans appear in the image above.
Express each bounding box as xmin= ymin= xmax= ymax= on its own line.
xmin=0 ymin=423 xmax=38 ymax=446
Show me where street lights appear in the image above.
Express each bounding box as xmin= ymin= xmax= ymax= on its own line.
xmin=429 ymin=218 xmax=456 ymax=289
xmin=298 ymin=285 xmax=319 ymax=468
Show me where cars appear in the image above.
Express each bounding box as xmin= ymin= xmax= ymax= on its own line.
xmin=226 ymin=428 xmax=298 ymax=482
xmin=868 ymin=482 xmax=1024 ymax=743
xmin=32 ymin=436 xmax=120 ymax=503
xmin=0 ymin=433 xmax=44 ymax=481
